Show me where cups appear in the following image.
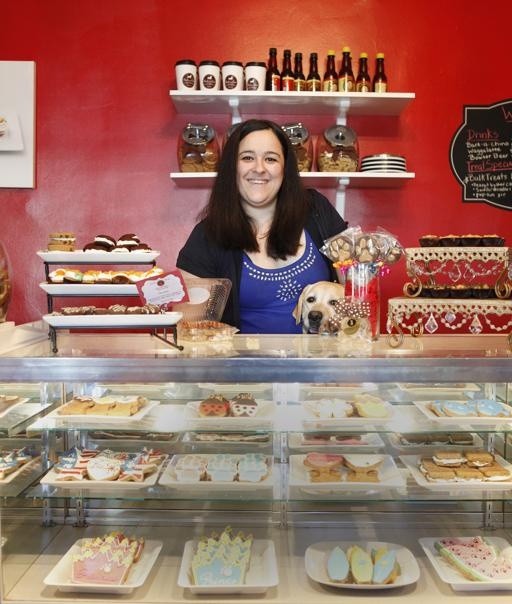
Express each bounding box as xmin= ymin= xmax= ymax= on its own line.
xmin=174 ymin=60 xmax=266 ymax=92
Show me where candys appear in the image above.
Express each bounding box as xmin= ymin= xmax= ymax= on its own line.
xmin=327 ymin=233 xmax=401 ymax=275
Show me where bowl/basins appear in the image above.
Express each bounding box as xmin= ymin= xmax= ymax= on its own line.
xmin=359 ymin=151 xmax=408 ymax=174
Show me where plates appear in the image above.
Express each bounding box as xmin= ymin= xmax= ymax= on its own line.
xmin=397 ymin=453 xmax=511 ymax=494
xmin=0 ymin=394 xmax=30 ymax=419
xmin=287 ymin=453 xmax=404 ymax=491
xmin=417 ymin=535 xmax=511 ymax=593
xmin=36 ymin=247 xmax=163 ymax=264
xmin=1 ymin=447 xmax=45 ymax=486
xmin=96 ymin=383 xmax=173 ymax=393
xmin=159 ymin=452 xmax=274 ymax=492
xmin=41 ymin=537 xmax=163 ymax=597
xmin=0 ymin=384 xmax=43 ymax=394
xmin=296 ymin=400 xmax=395 ymax=428
xmin=39 ymin=450 xmax=171 ymax=492
xmin=54 ymin=400 xmax=161 ymax=423
xmin=175 ymin=537 xmax=279 ymax=595
xmin=41 ymin=311 xmax=184 ymax=327
xmin=197 ymin=383 xmax=273 ymax=393
xmin=184 ymin=399 xmax=270 ymax=427
xmin=37 ymin=279 xmax=142 ymax=298
xmin=300 ymin=382 xmax=378 ymax=396
xmin=412 ymin=399 xmax=512 ymax=427
xmin=302 ymin=537 xmax=421 ymax=590
xmin=398 ymin=383 xmax=480 ymax=397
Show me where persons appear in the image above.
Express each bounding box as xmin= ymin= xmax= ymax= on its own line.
xmin=176 ymin=118 xmax=349 ymax=336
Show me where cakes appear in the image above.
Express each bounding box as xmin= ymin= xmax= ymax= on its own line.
xmin=189 ymin=523 xmax=255 ymax=586
xmin=71 ymin=531 xmax=146 ymax=585
xmin=303 ymin=454 xmax=386 ymax=483
xmin=61 ymin=304 xmax=162 ymax=314
xmin=427 ymin=398 xmax=512 ymax=417
xmin=47 ymin=233 xmax=152 ymax=254
xmin=420 ymin=234 xmax=504 ymax=247
xmin=433 ymin=535 xmax=511 ymax=582
xmin=49 ymin=266 xmax=165 ymax=284
xmin=408 ymin=283 xmax=501 ymax=299
xmin=198 ymin=393 xmax=258 ymax=417
xmin=325 ymin=544 xmax=399 ymax=585
xmin=174 ymin=454 xmax=268 ymax=483
xmin=417 ymin=451 xmax=509 ymax=483
xmin=61 ymin=395 xmax=148 ymax=417
xmin=303 ymin=394 xmax=391 ymax=418
xmin=0 ymin=443 xmax=34 ymax=479
xmin=0 ymin=394 xmax=19 ymax=412
xmin=55 ymin=447 xmax=163 ymax=482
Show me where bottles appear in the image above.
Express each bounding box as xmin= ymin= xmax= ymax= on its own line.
xmin=266 ymin=44 xmax=387 ymax=94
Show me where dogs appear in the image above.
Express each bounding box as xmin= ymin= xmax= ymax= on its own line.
xmin=292 ymin=280 xmax=346 ymax=359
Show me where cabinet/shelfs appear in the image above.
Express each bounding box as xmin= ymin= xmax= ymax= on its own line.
xmin=1 ymin=333 xmax=512 ymax=603
xmin=167 ymin=87 xmax=418 ymax=221
